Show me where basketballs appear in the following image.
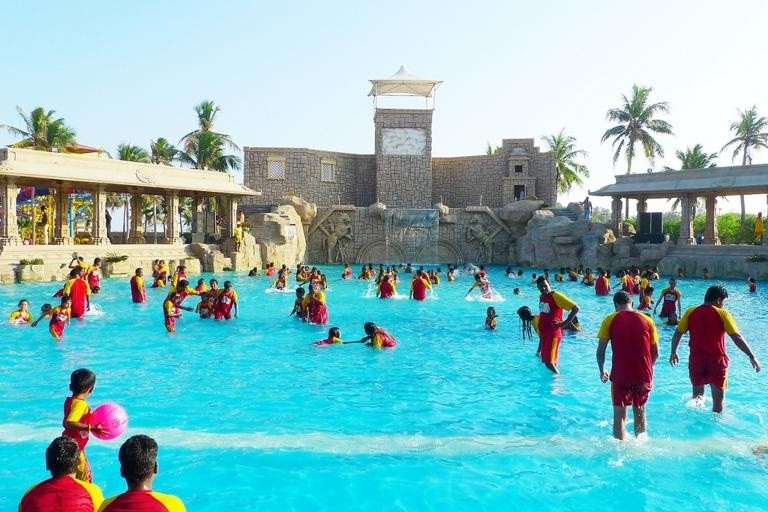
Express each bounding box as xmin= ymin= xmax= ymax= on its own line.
xmin=91 ymin=404 xmax=127 ymax=440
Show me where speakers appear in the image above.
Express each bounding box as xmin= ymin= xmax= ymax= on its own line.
xmin=191 ymin=212 xmax=220 ymax=244
xmin=639 ymin=213 xmax=663 ymax=243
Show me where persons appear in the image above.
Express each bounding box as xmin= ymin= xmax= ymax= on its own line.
xmin=63 ymin=368 xmax=112 ymax=482
xmin=17 ymin=435 xmax=105 ymax=512
xmin=752 ymin=212 xmax=764 ymax=245
xmin=669 ymin=284 xmax=761 ymax=418
xmin=234 ymin=222 xmax=243 ymax=251
xmin=236 ymin=210 xmax=245 ymax=225
xmin=215 ymin=214 xmax=224 ymax=240
xmin=105 ymin=210 xmax=117 ymax=243
xmin=597 ymin=290 xmax=659 ymax=442
xmin=535 ymin=275 xmax=582 ymax=376
xmin=580 ymin=196 xmax=594 ymax=221
xmin=99 ymin=434 xmax=188 ymax=512
xmin=39 ymin=205 xmax=50 ymax=246
